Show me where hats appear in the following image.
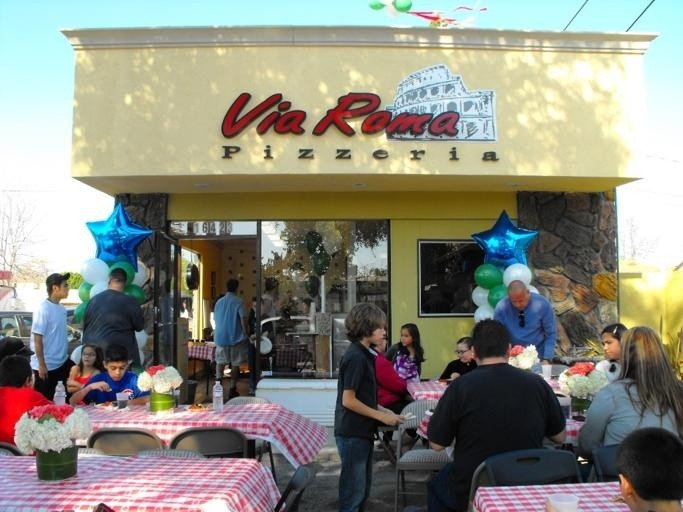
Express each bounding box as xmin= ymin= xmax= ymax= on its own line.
xmin=1 ymin=336 xmax=34 ymax=360
xmin=45 ymin=273 xmax=71 ymax=289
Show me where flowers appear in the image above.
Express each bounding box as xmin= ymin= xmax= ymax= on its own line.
xmin=506 ymin=343 xmax=542 ymax=374
xmin=557 ymin=361 xmax=611 ymax=398
xmin=136 ymin=365 xmax=184 ymax=393
xmin=12 ymin=404 xmax=92 ymax=455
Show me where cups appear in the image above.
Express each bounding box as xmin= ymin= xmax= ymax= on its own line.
xmin=169 ymin=390 xmax=181 ymax=410
xmin=541 ymin=365 xmax=552 ymax=383
xmin=548 ymin=492 xmax=581 ymax=512
xmin=116 ymin=392 xmax=128 ymax=408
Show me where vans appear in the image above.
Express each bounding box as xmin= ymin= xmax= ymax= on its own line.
xmin=261 ymin=318 xmax=352 ymax=370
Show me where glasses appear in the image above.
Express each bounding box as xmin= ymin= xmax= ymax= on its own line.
xmin=519 ymin=311 xmax=526 ymax=327
xmin=81 ymin=351 xmax=95 ymax=357
xmin=454 ymin=347 xmax=471 ymax=355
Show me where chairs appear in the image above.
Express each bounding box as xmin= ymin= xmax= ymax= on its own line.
xmin=530 ymin=364 xmax=571 ymax=379
xmin=168 ymin=426 xmax=248 ymax=459
xmin=225 ymin=396 xmax=276 ymax=482
xmin=87 ymin=428 xmax=165 ymax=455
xmin=392 ymin=398 xmax=457 ymax=512
xmin=470 ymin=448 xmax=584 ymax=501
xmin=591 ymin=443 xmax=624 ymax=481
xmin=276 ymin=460 xmax=319 ymax=511
xmin=264 ymin=345 xmax=311 ymax=379
xmin=372 ymin=422 xmax=404 ymax=466
xmin=0 ymin=441 xmax=23 ymax=456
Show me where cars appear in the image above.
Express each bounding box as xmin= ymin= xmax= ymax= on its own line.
xmin=0 ymin=311 xmax=81 ymax=354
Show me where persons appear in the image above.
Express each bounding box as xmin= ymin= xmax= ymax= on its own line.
xmin=248 ymin=296 xmax=273 ymax=371
xmin=335 ymin=304 xmax=404 ymax=512
xmin=494 ymin=280 xmax=555 ymax=366
xmin=29 ymin=272 xmax=72 ymax=403
xmin=439 ymin=337 xmax=478 ymax=381
xmin=80 ymin=267 xmax=144 ymax=373
xmin=69 ymin=345 xmax=151 ymax=406
xmin=425 ymin=319 xmax=567 ymax=512
xmin=369 ymin=332 xmax=419 ymax=450
xmin=383 ymin=323 xmax=424 ymax=385
xmin=261 ymin=278 xmax=279 ymax=337
xmin=595 ymin=323 xmax=628 ymax=380
xmin=579 ymin=326 xmax=683 ymax=483
xmin=66 ymin=342 xmax=107 ymax=405
xmin=614 ymin=427 xmax=683 ymax=512
xmin=0 ymin=356 xmax=55 ymax=455
xmin=214 ymin=279 xmax=249 ymax=399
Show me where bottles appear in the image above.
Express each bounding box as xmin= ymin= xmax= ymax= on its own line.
xmin=55 ymin=381 xmax=66 ymax=405
xmin=212 ymin=381 xmax=223 ymax=411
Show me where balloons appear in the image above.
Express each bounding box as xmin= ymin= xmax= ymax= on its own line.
xmin=78 ymin=281 xmax=93 ymax=302
xmin=529 ymin=284 xmax=540 ymax=293
xmin=80 ymin=258 xmax=109 ymax=285
xmin=322 ymin=229 xmax=342 ymax=255
xmin=123 ymin=285 xmax=146 ymax=305
xmin=503 ymin=262 xmax=533 ymax=289
xmin=303 ymin=275 xmax=320 ymax=299
xmin=471 ymin=286 xmax=489 ymax=307
xmin=474 ymin=263 xmax=503 ymax=289
xmin=473 ymin=305 xmax=495 ymax=323
xmin=109 ymin=262 xmax=135 ymax=286
xmin=487 ymin=284 xmax=508 ymax=309
xmin=305 ymin=230 xmax=322 ymax=254
xmin=90 ymin=281 xmax=108 ymax=299
xmin=75 ymin=301 xmax=89 ymax=321
xmin=311 ymin=250 xmax=330 ymax=276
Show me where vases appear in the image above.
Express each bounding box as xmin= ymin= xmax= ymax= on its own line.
xmin=569 ymin=394 xmax=593 ymax=420
xmin=34 ymin=445 xmax=80 ymax=478
xmin=150 ymin=388 xmax=178 ymax=413
